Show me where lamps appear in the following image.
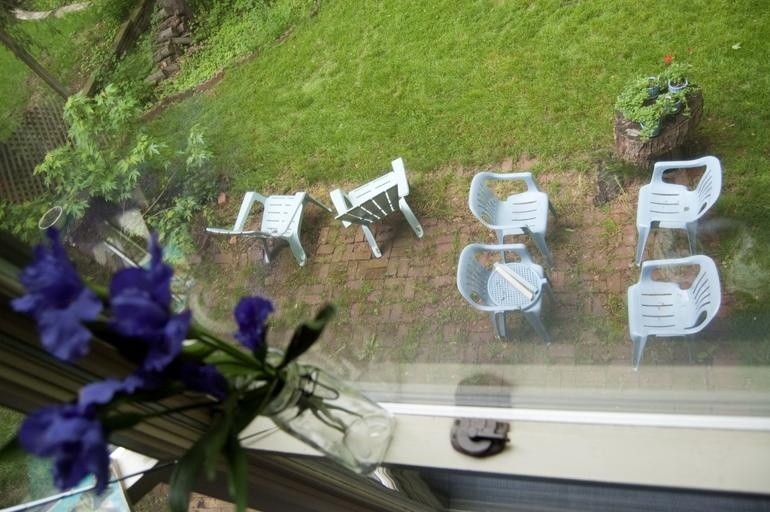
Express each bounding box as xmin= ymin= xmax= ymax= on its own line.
xmin=329 ymin=157 xmax=423 ymax=258
xmin=456 ymin=243 xmax=556 ymax=346
xmin=206 ymin=190 xmax=331 ymax=266
xmin=633 ymin=156 xmax=722 ymax=268
xmin=468 ymin=171 xmax=559 ymax=266
xmin=627 ymin=255 xmax=721 ymax=372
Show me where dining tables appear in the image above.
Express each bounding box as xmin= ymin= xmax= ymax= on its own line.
xmin=615 ymin=64 xmax=698 ymax=141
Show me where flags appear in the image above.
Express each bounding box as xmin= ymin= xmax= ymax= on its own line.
xmin=241 ymin=349 xmax=398 ymax=478
xmin=38 ymin=206 xmax=66 ymax=233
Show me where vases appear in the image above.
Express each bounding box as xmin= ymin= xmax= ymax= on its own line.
xmin=8 ymin=229 xmax=361 ymax=512
